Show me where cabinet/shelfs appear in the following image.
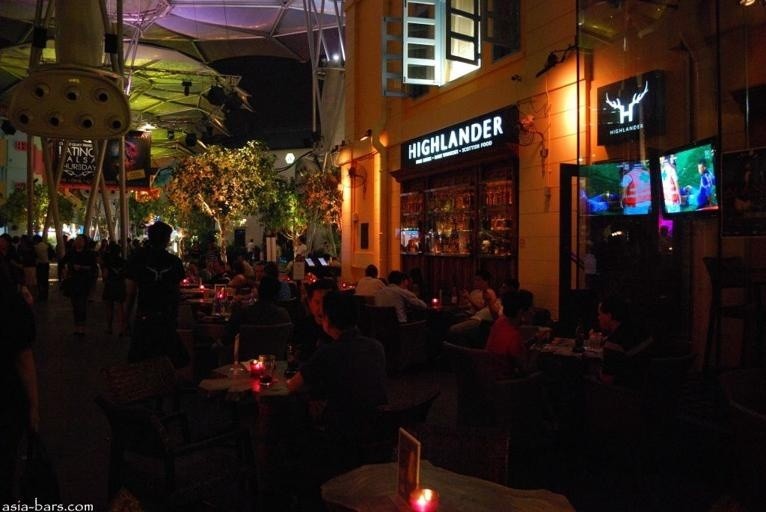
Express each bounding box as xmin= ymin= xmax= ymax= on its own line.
xmin=400 ymin=162 xmax=517 ymax=294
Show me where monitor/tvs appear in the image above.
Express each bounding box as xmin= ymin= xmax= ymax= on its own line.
xmin=654 ymin=135 xmax=724 ymax=221
xmin=577 ymin=156 xmax=654 ymax=224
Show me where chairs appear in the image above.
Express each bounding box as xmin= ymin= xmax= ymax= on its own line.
xmin=92 ymin=392 xmax=254 ymax=512
xmin=702 ymin=255 xmax=766 ymax=365
xmin=725 ymin=367 xmax=766 ymax=455
xmin=362 ymin=303 xmax=429 ymax=377
xmin=440 ymin=339 xmax=546 ymax=486
xmin=313 ymin=391 xmax=441 ymax=486
xmin=263 ymin=296 xmax=298 ymax=324
xmin=237 ymin=323 xmax=293 ymax=362
xmin=583 ymin=338 xmax=705 ymax=440
xmin=287 ymin=280 xmax=298 ymax=297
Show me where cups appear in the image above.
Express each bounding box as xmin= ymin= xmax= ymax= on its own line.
xmin=259 ymin=361 xmax=273 ymax=386
xmin=287 ymin=344 xmax=298 ymax=367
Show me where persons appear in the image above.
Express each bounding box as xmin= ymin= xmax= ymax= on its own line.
xmin=680 ymin=160 xmax=713 ymax=208
xmin=247 ymin=238 xmax=255 ymax=260
xmin=450 ymin=282 xmax=499 ymax=332
xmin=0 ymin=232 xmax=142 ymax=336
xmin=597 ymin=297 xmax=654 ymax=389
xmin=248 ymin=261 xmax=291 ymax=300
xmin=0 ymin=268 xmax=41 ymax=510
xmin=661 ymin=154 xmax=681 ymax=213
xmin=287 ymin=292 xmax=385 ymax=437
xmin=618 ymin=162 xmax=651 ymax=214
xmin=292 ymin=278 xmax=340 ymax=368
xmin=355 ymin=264 xmax=385 ymax=306
xmin=484 ymin=289 xmax=537 ymax=376
xmin=376 ymin=271 xmax=427 ymax=324
xmin=296 ymin=241 xmax=307 ymax=261
xmin=463 ymin=272 xmax=492 ymax=311
xmin=220 ymin=276 xmax=293 ymax=362
xmin=122 ymin=220 xmax=186 ymax=364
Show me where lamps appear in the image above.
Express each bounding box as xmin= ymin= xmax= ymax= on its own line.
xmin=535 ymin=44 xmax=576 ymax=78
xmin=330 ymin=145 xmax=339 ymax=155
xmin=359 ymin=129 xmax=371 ymax=142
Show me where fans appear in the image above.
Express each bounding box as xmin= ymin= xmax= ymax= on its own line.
xmin=336 ymin=161 xmax=368 ymax=192
xmin=502 ymin=110 xmax=548 ymax=159
